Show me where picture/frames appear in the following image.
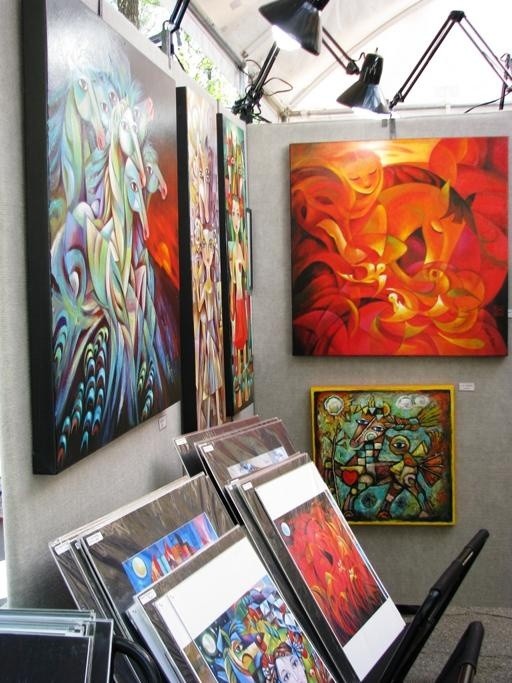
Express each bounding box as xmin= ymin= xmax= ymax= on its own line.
xmin=310 ymin=384 xmax=457 ymax=527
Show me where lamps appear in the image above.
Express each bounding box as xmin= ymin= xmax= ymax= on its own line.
xmin=336 ymin=53 xmax=390 ymax=118
xmin=258 ymin=0 xmax=329 ymax=56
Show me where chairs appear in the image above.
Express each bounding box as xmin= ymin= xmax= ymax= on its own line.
xmin=379 ymin=528 xmax=491 ymax=682
xmin=110 ymin=634 xmax=163 ymax=682
xmin=435 ymin=620 xmax=485 ymax=682
